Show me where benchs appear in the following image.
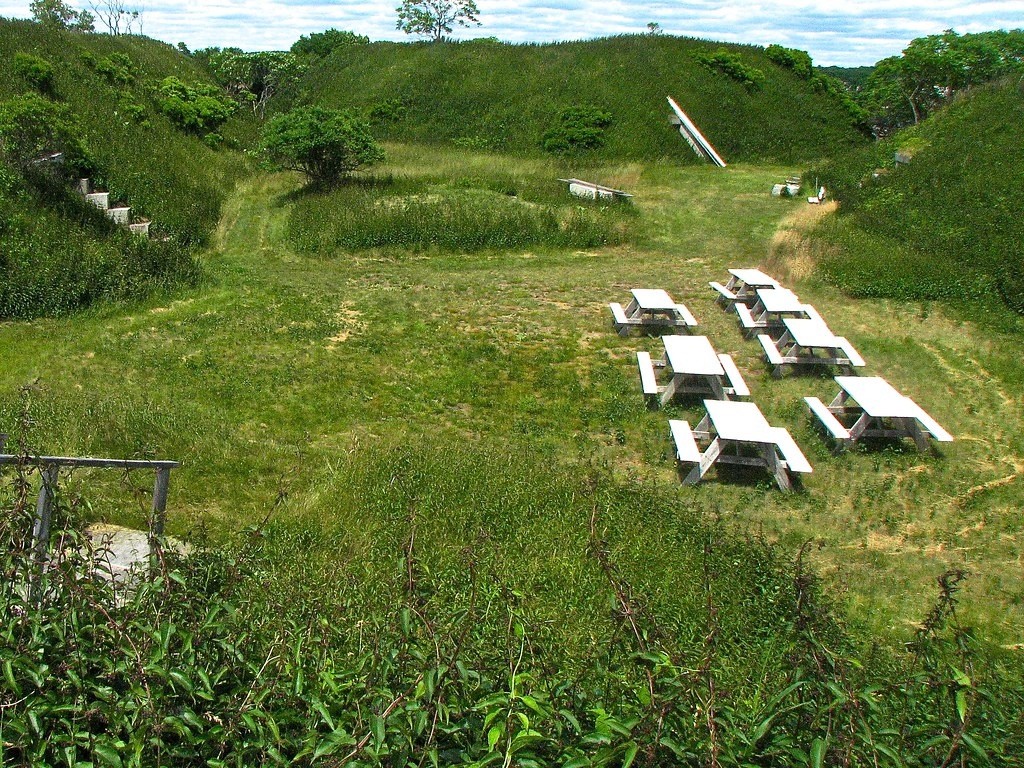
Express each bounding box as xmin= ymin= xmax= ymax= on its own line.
xmin=675 ymin=304 xmax=698 ymax=327
xmin=717 ymin=354 xmax=751 ymax=397
xmin=773 ymin=285 xmax=799 ymax=300
xmin=735 ymin=303 xmax=757 ymax=329
xmin=770 ymin=426 xmax=814 ymax=473
xmin=836 ymin=336 xmax=866 ymax=367
xmin=637 ymin=351 xmax=664 ymax=395
xmin=669 ymin=419 xmax=703 ymax=463
xmin=904 ymin=396 xmax=954 ymax=442
xmin=802 ymin=304 xmax=827 ymax=326
xmin=709 ymin=281 xmax=738 ymax=301
xmin=609 ymin=302 xmax=630 ymax=325
xmin=803 ymin=396 xmax=851 ymax=440
xmin=757 ymin=334 xmax=786 ymax=365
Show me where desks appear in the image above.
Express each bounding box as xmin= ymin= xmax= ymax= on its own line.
xmin=812 ymin=376 xmax=939 ymax=458
xmin=652 ymin=335 xmax=731 ymax=408
xmin=617 ymin=288 xmax=693 ymax=335
xmin=762 ymin=319 xmax=857 ymax=379
xmin=737 ymin=289 xmax=806 ymax=340
xmin=679 ymin=399 xmax=796 ymax=495
xmin=714 ymin=268 xmax=780 ymax=314
